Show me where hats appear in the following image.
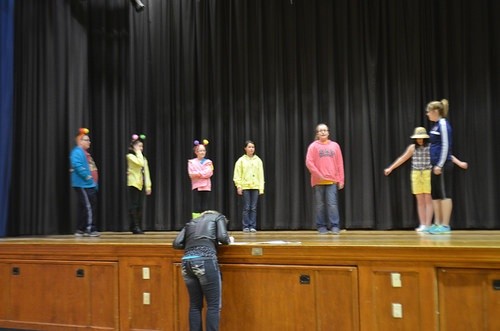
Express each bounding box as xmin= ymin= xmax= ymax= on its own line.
xmin=411 ymin=127 xmax=431 ymax=138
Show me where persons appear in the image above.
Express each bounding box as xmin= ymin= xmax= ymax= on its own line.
xmin=423 ymin=99 xmax=453 ymax=235
xmin=173 ymin=211 xmax=234 ymax=331
xmin=384 ymin=127 xmax=467 ymax=232
xmin=233 ymin=141 xmax=264 ymax=233
xmin=69 ymin=127 xmax=100 ymax=238
xmin=306 ymin=124 xmax=344 ymax=235
xmin=127 ymin=134 xmax=152 ymax=235
xmin=188 ymin=139 xmax=214 ymax=219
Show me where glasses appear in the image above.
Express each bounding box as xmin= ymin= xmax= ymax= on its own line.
xmin=318 ymin=129 xmax=329 ymax=133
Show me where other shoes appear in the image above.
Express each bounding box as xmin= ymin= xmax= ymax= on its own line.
xmin=331 ymin=226 xmax=340 ymax=234
xmin=75 ymin=230 xmax=84 ymax=237
xmin=317 ymin=226 xmax=330 ymax=234
xmin=416 ymin=225 xmax=425 ymax=232
xmin=132 ymin=227 xmax=146 ymax=235
xmin=424 ymin=224 xmax=439 ymax=233
xmin=243 ymin=227 xmax=250 ymax=232
xmin=431 ymin=225 xmax=450 ymax=235
xmin=250 ymin=228 xmax=256 ymax=233
xmin=84 ymin=230 xmax=100 ymax=237
